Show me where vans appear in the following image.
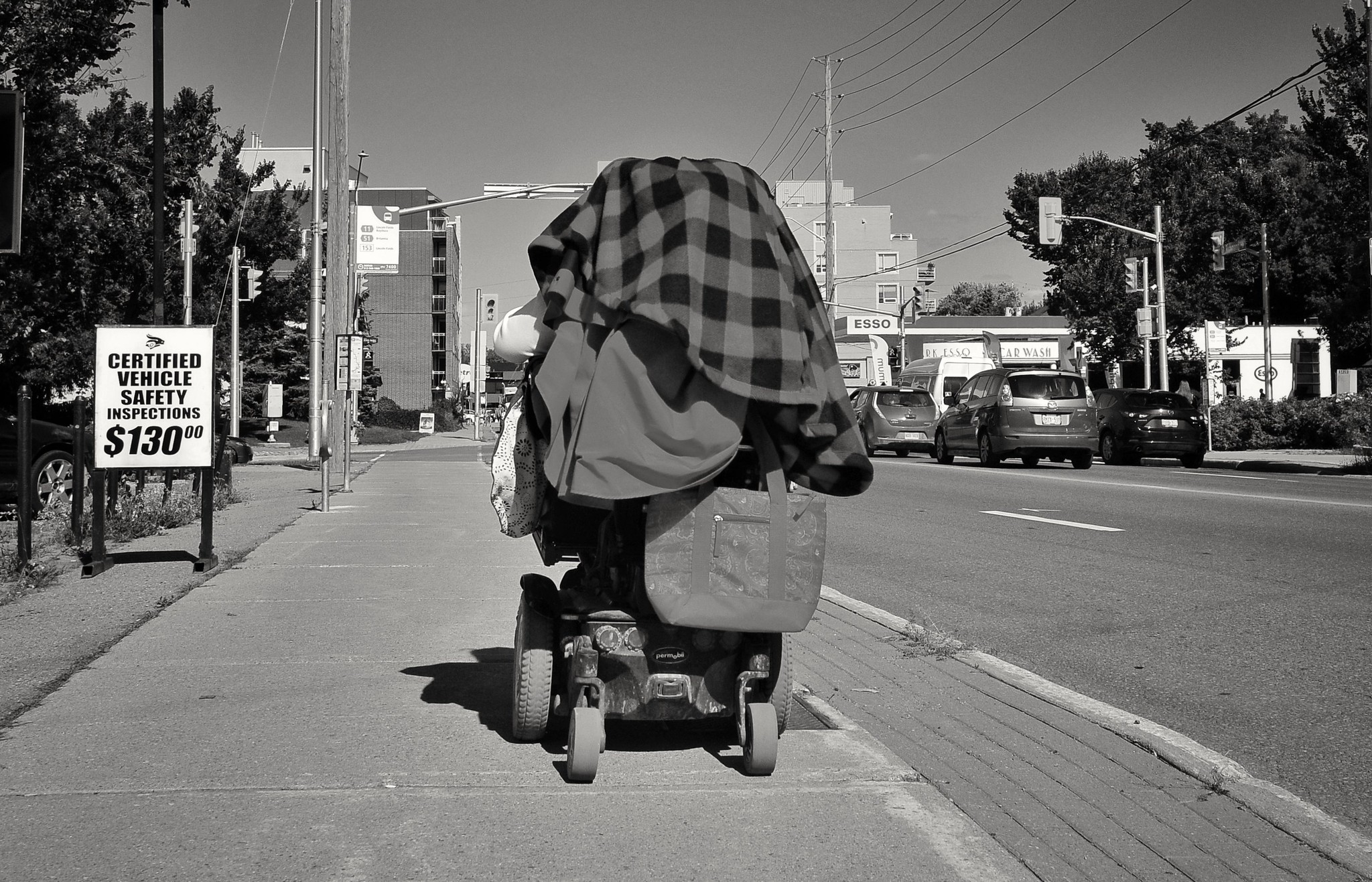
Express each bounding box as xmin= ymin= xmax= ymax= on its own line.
xmin=894 ymin=357 xmax=997 ymax=413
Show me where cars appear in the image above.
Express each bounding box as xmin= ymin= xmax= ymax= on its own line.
xmin=462 ymin=409 xmax=475 ymax=425
xmin=214 ymin=432 xmax=255 ymax=465
xmin=847 ymin=386 xmax=943 ymax=458
xmin=0 ymin=412 xmax=95 ymax=517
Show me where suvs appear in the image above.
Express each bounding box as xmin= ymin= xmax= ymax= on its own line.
xmin=935 ymin=367 xmax=1098 ymax=470
xmin=1091 ymin=388 xmax=1206 ymax=469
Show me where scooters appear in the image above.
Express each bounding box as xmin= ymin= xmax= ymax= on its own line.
xmin=511 ymin=159 xmax=830 ymax=783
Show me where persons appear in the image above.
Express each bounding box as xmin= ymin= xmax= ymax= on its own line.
xmin=1259 ymin=388 xmax=1265 ymax=400
xmin=492 ymin=289 xmax=556 ymax=363
xmin=1175 ymin=380 xmax=1202 ymax=409
xmin=499 ymin=409 xmax=506 ymax=432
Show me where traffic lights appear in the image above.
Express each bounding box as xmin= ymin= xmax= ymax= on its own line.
xmin=1124 ymin=257 xmax=1137 ymax=292
xmin=482 ymin=294 xmax=498 ymax=324
xmin=181 ymin=222 xmax=200 ymax=238
xmin=912 ymin=282 xmax=925 ymax=312
xmin=1210 ymin=230 xmax=1225 ymax=270
xmin=888 ymin=347 xmax=898 ymax=368
xmin=248 ymin=267 xmax=263 ymax=302
xmin=360 ymin=272 xmax=370 ymax=296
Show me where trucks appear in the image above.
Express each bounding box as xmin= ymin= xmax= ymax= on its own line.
xmin=502 ymin=387 xmax=519 ymax=404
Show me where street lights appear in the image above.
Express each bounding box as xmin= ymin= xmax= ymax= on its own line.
xmin=435 ymin=374 xmax=451 ymax=400
xmin=348 ymin=149 xmax=369 ymax=445
